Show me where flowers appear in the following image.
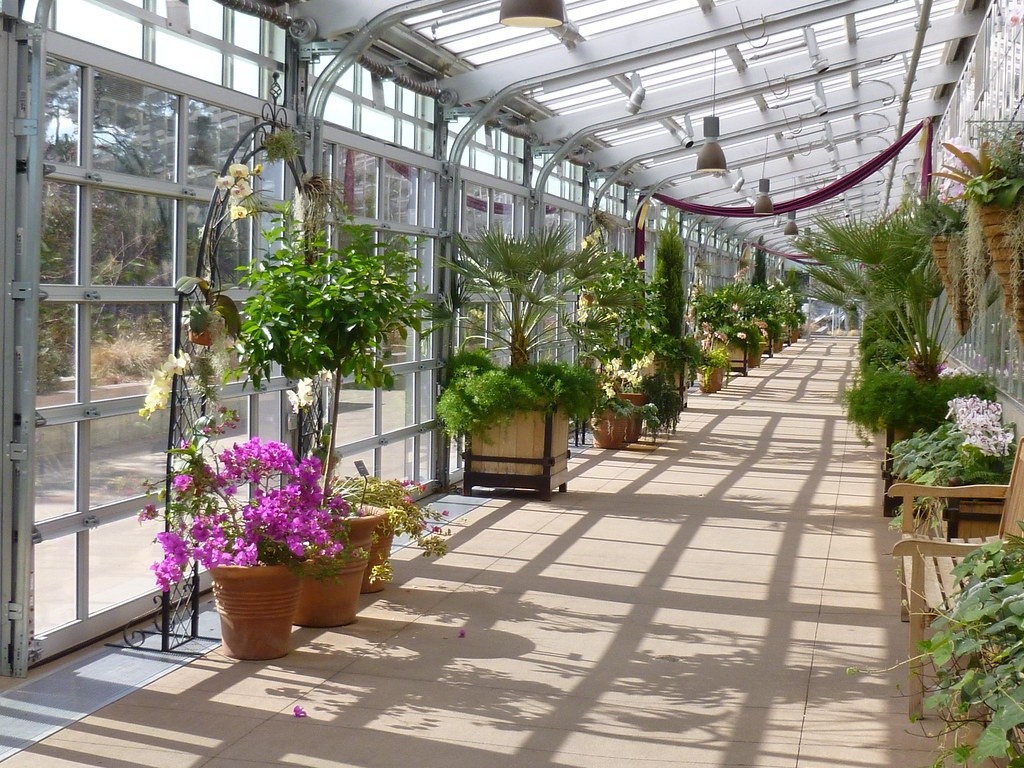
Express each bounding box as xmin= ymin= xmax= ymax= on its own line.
xmin=138 ymin=435 xmax=353 ymax=593
xmin=326 ymin=470 xmax=472 ymax=562
xmin=946 ymin=396 xmax=1014 ymax=468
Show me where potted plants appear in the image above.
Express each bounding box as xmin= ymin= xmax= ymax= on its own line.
xmin=433 ymin=209 xmax=811 ymax=508
xmin=181 ymin=306 xmax=230 ymax=381
xmin=926 ymin=136 xmax=1024 ymax=348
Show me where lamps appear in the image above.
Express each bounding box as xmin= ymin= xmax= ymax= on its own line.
xmin=498 ymin=0 xmax=860 ymax=257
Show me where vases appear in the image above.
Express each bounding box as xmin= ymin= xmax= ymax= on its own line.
xmin=363 ymin=509 xmax=396 ymax=594
xmin=294 ymin=502 xmax=388 ymax=627
xmin=208 ymin=565 xmax=303 ymax=661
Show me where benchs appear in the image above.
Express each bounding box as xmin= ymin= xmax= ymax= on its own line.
xmin=890 ymin=438 xmax=1024 ymax=720
